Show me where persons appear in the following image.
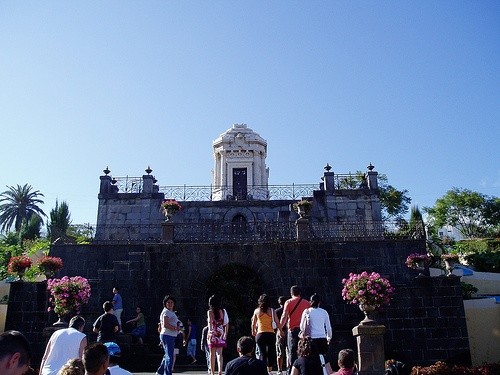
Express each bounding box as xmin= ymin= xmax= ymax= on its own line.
xmin=224 ymin=336 xmax=269 ymax=375
xmin=224 ymin=317 xmax=251 ymax=358
xmin=330 ymin=348 xmax=359 ymax=375
xmin=297 ymin=292 xmax=333 ymax=369
xmin=290 ymin=336 xmax=325 ymax=375
xmin=273 ymin=295 xmax=291 ymax=375
xmin=42 ymin=315 xmax=88 ymax=375
xmin=154 ymin=294 xmax=181 ymax=375
xmin=185 ymin=317 xmax=199 ymax=365
xmin=280 ymin=284 xmax=311 ymax=367
xmin=252 ymin=292 xmax=285 ymax=375
xmin=206 ymin=294 xmax=230 ymax=375
xmin=199 ymin=317 xmax=214 ymax=374
xmin=62 ymin=286 xmax=147 ymax=375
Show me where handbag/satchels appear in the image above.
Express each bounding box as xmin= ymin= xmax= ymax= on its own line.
xmin=271 ymin=308 xmax=277 ymax=329
xmin=298 ymin=309 xmax=311 ymax=338
xmin=223 ymin=308 xmax=229 ymax=326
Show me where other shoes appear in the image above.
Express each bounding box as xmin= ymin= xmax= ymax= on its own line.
xmin=277 ymin=372 xmax=283 ymax=375
xmin=268 ymin=372 xmax=273 ymax=375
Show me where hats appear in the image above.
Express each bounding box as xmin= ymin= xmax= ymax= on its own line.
xmin=103 ymin=342 xmax=121 ymax=357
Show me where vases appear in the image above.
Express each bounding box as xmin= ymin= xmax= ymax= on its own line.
xmin=165 ymin=209 xmax=175 ymax=223
xmin=444 ymin=259 xmax=456 ymax=274
xmin=45 ymin=269 xmax=51 ymax=278
xmin=53 ymin=317 xmax=68 ymax=325
xmin=415 ymin=261 xmax=425 ymax=275
xmin=298 ymin=205 xmax=306 ymax=219
xmin=18 ymin=269 xmax=24 ymax=283
xmin=359 ymin=305 xmax=377 ymax=325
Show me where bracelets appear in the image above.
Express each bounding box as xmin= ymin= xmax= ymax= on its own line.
xmin=174 ymin=327 xmax=177 ymax=330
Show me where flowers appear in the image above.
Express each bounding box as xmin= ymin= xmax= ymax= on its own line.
xmin=8 ymin=255 xmax=33 ymax=272
xmin=161 ymin=200 xmax=182 ymax=216
xmin=37 ymin=256 xmax=63 ymax=275
xmin=293 ymin=199 xmax=314 ymax=214
xmin=47 ymin=275 xmax=91 ymax=317
xmin=341 ymin=271 xmax=395 ymax=311
xmin=441 ymin=254 xmax=459 ymax=261
xmin=405 ymin=253 xmax=431 ymax=270
